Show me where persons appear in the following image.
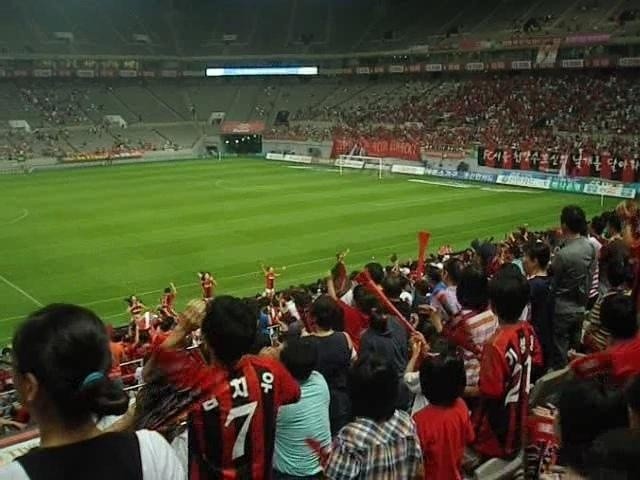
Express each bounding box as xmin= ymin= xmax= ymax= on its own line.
xmin=251 ymin=6 xmax=640 ymax=184
xmin=273 ymin=197 xmax=640 ymax=480
xmin=0 ymin=75 xmax=172 ymax=160
xmin=1 ymin=263 xmax=301 ymax=479
